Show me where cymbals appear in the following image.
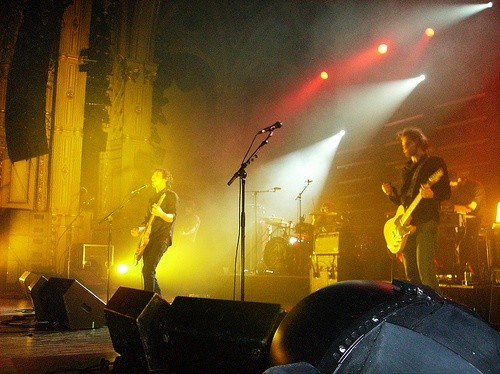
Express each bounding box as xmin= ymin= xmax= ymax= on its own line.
xmin=308 ymin=210 xmax=339 ymax=217
xmin=267 ymin=217 xmax=292 ymax=230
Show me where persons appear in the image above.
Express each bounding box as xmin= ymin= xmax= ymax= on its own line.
xmin=382 ymin=128 xmax=451 ymax=294
xmin=180 ymin=200 xmax=199 ymax=243
xmin=131 ymin=168 xmax=179 ymax=293
xmin=447 ymin=168 xmax=481 ymax=285
xmin=312 ymin=204 xmax=331 ymax=232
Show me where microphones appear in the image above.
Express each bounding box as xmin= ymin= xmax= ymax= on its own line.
xmin=258 ymin=121 xmax=283 ymax=134
xmin=131 ymin=185 xmax=148 ymax=193
xmin=272 ymin=187 xmax=282 ymax=190
xmin=82 ymin=197 xmax=94 ymax=205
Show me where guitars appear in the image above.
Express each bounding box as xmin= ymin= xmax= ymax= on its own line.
xmin=382 ymin=166 xmax=444 ymax=256
xmin=132 ymin=190 xmax=168 ymax=263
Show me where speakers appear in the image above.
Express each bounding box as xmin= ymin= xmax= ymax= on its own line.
xmin=158 ymin=296 xmax=288 ymax=374
xmin=488 ymin=285 xmax=500 ymax=326
xmin=309 ymin=232 xmax=348 ymax=295
xmin=19 ymin=271 xmax=107 ymax=330
xmin=5 ymin=0 xmax=65 ymax=162
xmin=104 ymin=286 xmax=171 ymax=372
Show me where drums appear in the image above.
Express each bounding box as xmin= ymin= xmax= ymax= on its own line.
xmin=264 ymin=236 xmax=293 ymax=273
xmin=293 ymin=222 xmax=316 ymax=241
